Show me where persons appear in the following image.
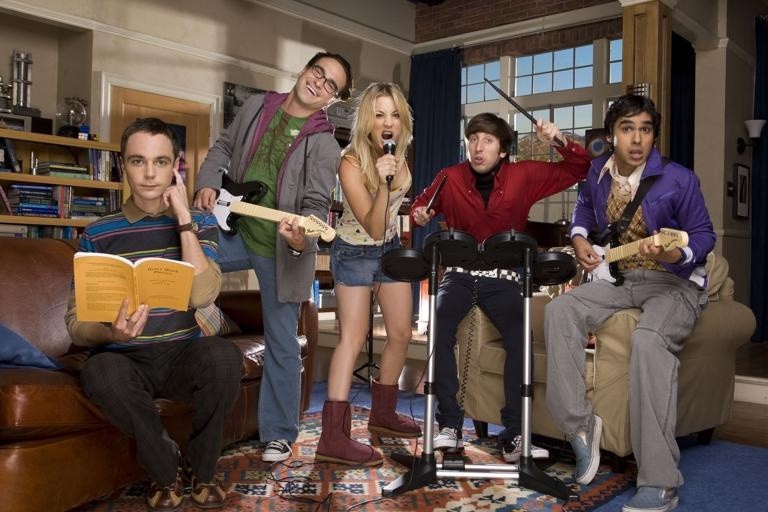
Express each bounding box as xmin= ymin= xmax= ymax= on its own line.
xmin=67 ymin=116 xmax=243 ymax=511
xmin=190 ymin=53 xmax=354 ymax=460
xmin=316 ymin=83 xmax=425 ymax=467
xmin=540 ymin=93 xmax=716 ymax=511
xmin=411 ymin=110 xmax=593 ymax=462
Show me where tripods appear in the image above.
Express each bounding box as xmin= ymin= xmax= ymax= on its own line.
xmin=353 ymin=288 xmax=380 ymax=390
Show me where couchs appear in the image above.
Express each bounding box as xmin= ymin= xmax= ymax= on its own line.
xmin=0 ymin=235 xmax=318 ymax=511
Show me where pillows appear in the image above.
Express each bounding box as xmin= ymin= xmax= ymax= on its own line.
xmin=1 ymin=324 xmax=62 ymax=373
xmin=193 ymin=302 xmax=243 ymax=340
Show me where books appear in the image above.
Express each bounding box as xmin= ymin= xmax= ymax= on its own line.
xmin=1 ymin=136 xmax=125 ymax=240
xmin=72 ymin=250 xmax=197 ymax=326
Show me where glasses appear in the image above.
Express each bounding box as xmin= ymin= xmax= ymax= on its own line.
xmin=312 ymin=65 xmax=338 ymax=95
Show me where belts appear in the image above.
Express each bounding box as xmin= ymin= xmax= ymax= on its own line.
xmin=444 ymin=265 xmax=524 ymax=283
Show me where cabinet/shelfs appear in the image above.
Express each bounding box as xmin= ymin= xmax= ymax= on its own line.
xmin=0 ymin=128 xmax=132 ymax=228
xmin=313 ymin=123 xmax=417 ymax=313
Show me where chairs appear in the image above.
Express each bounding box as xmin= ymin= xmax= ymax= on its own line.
xmin=454 ymin=250 xmax=757 ymax=475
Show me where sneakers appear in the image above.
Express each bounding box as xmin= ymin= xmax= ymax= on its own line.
xmin=500 ymin=435 xmax=550 ymax=463
xmin=622 ymin=485 xmax=679 ymax=512
xmin=192 ymin=476 xmax=225 ymax=508
xmin=431 ymin=427 xmax=464 ymax=453
xmin=568 ymin=416 xmax=602 ymax=484
xmin=261 ymin=440 xmax=291 ymax=462
xmin=145 ymin=473 xmax=182 ymax=512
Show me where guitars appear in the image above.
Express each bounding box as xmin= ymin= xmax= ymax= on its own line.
xmin=191 ymin=172 xmax=337 ymax=243
xmin=547 ymin=228 xmax=689 ymax=299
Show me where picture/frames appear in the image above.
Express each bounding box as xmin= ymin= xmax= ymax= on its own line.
xmin=731 ymin=162 xmax=751 ymax=221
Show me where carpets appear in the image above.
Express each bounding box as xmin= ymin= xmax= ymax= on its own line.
xmin=76 ymin=402 xmax=639 ymax=510
xmin=71 ymin=377 xmax=768 ymax=511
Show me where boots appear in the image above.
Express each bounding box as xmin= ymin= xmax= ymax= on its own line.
xmin=367 ymin=376 xmax=420 ymax=438
xmin=314 ymin=401 xmax=382 ymax=468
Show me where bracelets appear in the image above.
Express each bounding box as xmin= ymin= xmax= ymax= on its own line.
xmin=288 ymin=246 xmax=303 ymax=256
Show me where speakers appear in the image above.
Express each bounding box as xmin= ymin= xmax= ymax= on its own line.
xmin=585 ymin=128 xmax=609 ymax=158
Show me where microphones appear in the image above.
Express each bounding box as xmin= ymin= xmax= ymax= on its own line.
xmin=383 ymin=139 xmax=396 ymax=183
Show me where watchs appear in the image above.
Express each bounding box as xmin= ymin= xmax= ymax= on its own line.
xmin=176 ymin=221 xmax=199 ymax=233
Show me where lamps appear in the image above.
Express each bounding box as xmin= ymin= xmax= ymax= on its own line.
xmin=736 ymin=119 xmax=767 ymax=155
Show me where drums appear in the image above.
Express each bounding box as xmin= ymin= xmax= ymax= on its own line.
xmin=381 ymin=248 xmax=431 ymax=282
xmin=481 ymin=232 xmax=538 ymax=269
xmin=422 ymin=229 xmax=477 ymax=268
xmin=532 ymin=252 xmax=577 ymax=286
